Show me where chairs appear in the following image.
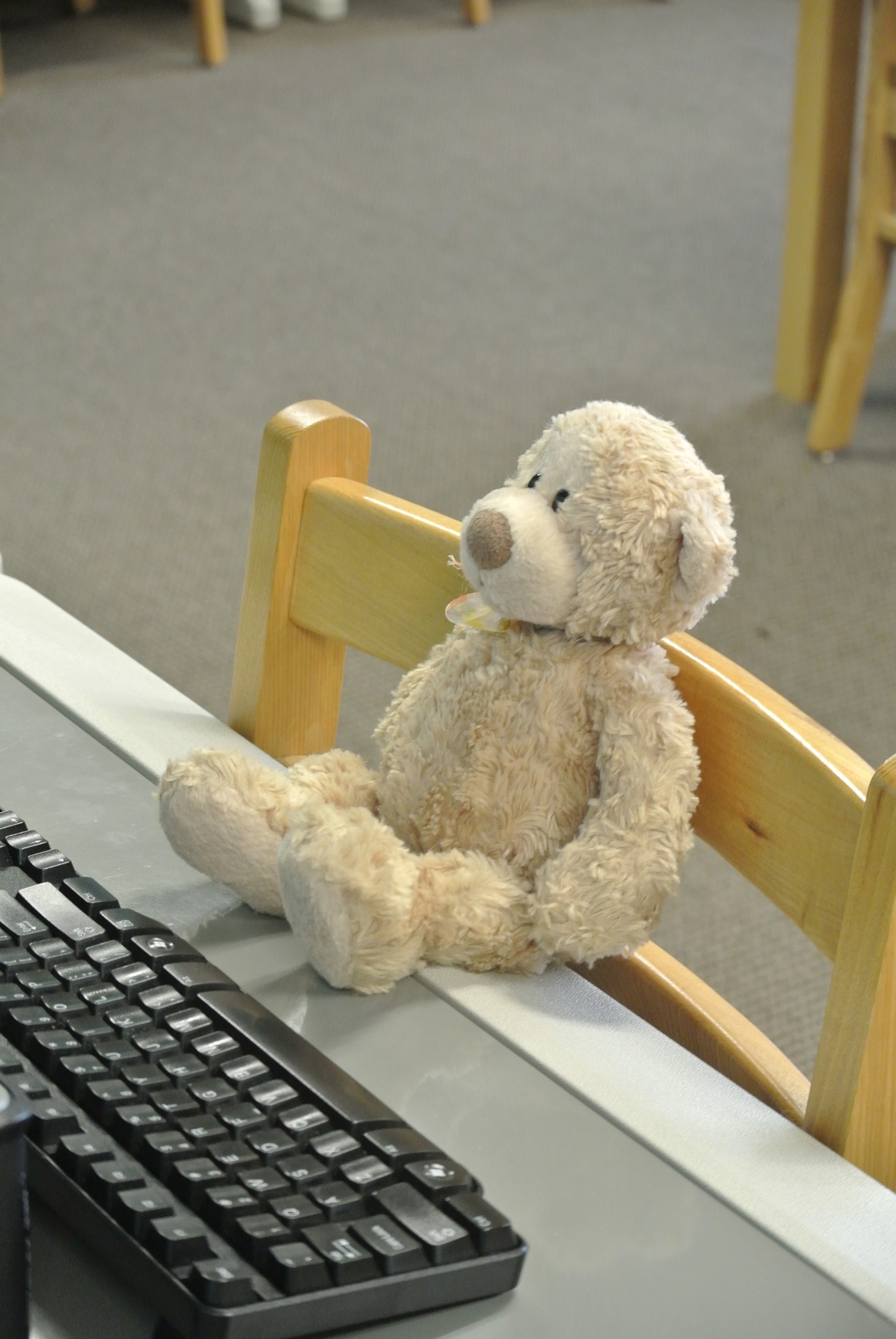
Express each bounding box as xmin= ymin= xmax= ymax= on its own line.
xmin=232 ymin=398 xmax=896 ymax=1194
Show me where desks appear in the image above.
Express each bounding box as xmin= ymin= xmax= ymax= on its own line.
xmin=0 ymin=574 xmax=895 ymax=1339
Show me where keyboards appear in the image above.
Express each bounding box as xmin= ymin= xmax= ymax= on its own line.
xmin=0 ymin=803 xmax=527 ymax=1339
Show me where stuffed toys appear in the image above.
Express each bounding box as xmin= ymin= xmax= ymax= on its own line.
xmin=159 ymin=399 xmax=737 ymax=994
